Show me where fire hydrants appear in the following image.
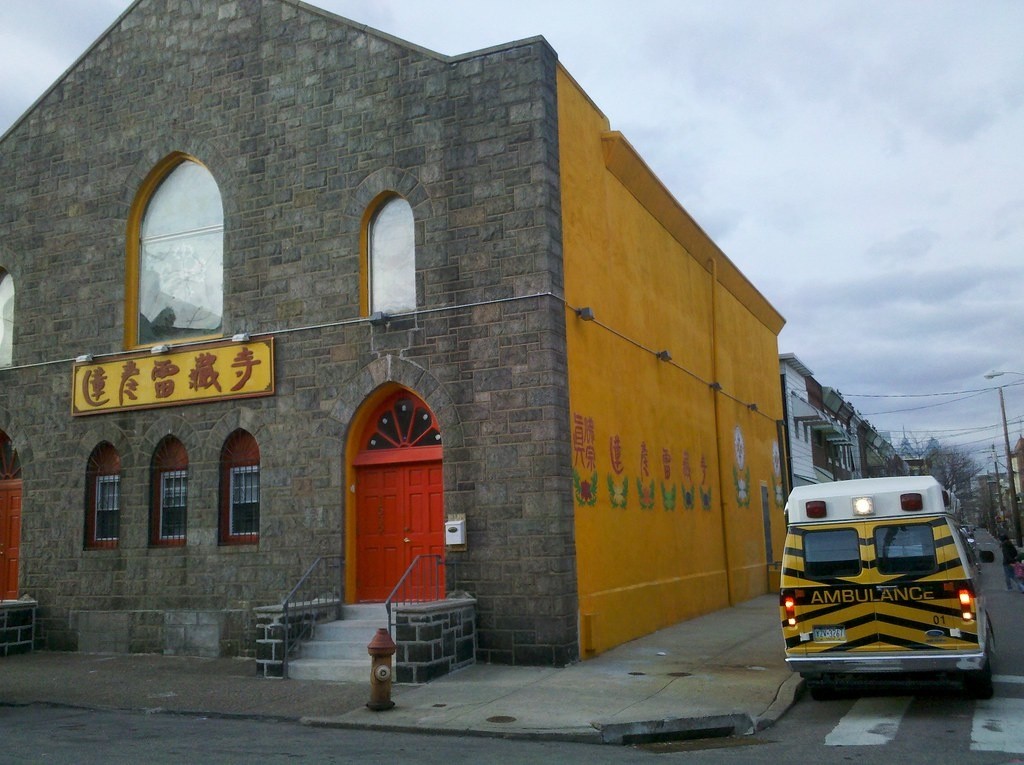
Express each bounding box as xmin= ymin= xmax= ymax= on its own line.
xmin=363 ymin=624 xmax=396 ymax=711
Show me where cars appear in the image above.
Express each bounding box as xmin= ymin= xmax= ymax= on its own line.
xmin=960 ymin=522 xmax=979 ymax=538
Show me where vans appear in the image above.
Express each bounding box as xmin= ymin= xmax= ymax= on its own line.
xmin=774 ymin=472 xmax=995 ymax=699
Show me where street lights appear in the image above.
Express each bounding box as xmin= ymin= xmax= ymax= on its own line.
xmin=983 ymin=370 xmax=1024 ymax=378
xmin=990 ymin=450 xmax=1008 ymax=521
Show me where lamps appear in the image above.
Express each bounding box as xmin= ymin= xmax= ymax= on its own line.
xmin=75 ymin=355 xmax=93 ymax=363
xmin=369 ymin=311 xmax=386 ymax=326
xmin=151 ymin=346 xmax=167 ymax=352
xmin=710 ymin=382 xmax=722 ymax=391
xmin=575 ymin=306 xmax=593 ymax=320
xmin=748 ymin=404 xmax=758 ymax=411
xmin=232 ymin=334 xmax=249 ymax=341
xmin=658 ymin=350 xmax=671 ymax=362
